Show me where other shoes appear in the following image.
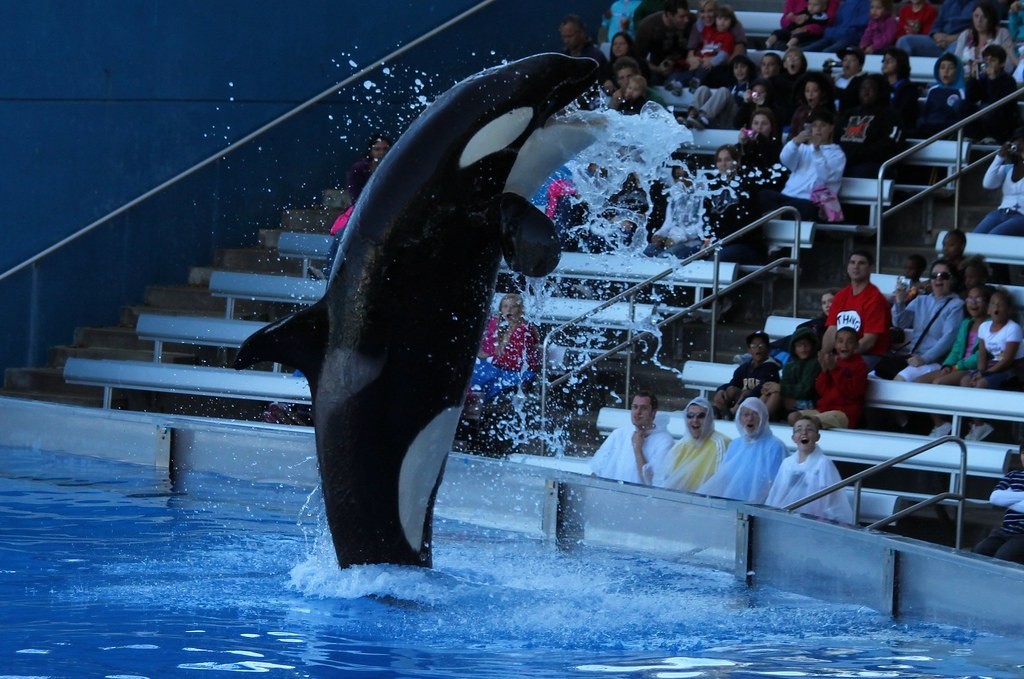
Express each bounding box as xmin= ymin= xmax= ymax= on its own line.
xmin=688 ymin=78 xmax=701 ymax=94
xmin=676 ymin=116 xmax=692 ymax=128
xmin=775 ymin=42 xmax=789 ymax=52
xmin=686 ymin=114 xmax=708 ymax=130
xmin=288 ymin=412 xmax=307 ymax=425
xmin=270 ymin=402 xmax=283 ymax=422
xmin=895 ymin=410 xmax=909 ymax=427
xmin=275 ymin=408 xmax=293 ymax=425
xmin=262 ymin=412 xmax=274 ymax=422
xmin=757 ymin=41 xmax=768 ymax=51
xmin=671 ymin=82 xmax=682 ymax=96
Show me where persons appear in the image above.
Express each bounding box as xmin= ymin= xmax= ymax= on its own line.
xmin=332 ymin=0 xmax=1024 ymax=566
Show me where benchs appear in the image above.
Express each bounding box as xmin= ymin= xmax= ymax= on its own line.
xmin=63 ymin=0 xmax=1024 ymax=527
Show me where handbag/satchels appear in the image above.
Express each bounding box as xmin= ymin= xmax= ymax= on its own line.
xmin=811 ymin=187 xmax=844 ymax=223
xmin=874 ymin=353 xmax=912 ymax=380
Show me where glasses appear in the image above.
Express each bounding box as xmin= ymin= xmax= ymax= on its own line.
xmin=687 ymin=412 xmax=706 ymax=420
xmin=882 ymin=57 xmax=897 ymax=64
xmin=966 ymin=295 xmax=985 ymax=303
xmin=740 ymin=410 xmax=759 ymax=418
xmin=749 ymin=342 xmax=765 ymax=349
xmin=786 ymin=56 xmax=801 ymax=62
xmin=929 ymin=271 xmax=952 ymax=280
xmin=793 ymin=427 xmax=817 ymax=435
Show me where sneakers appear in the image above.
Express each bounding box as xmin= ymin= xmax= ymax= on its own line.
xmin=965 ymin=423 xmax=994 ymax=441
xmin=930 ymin=423 xmax=952 ymax=438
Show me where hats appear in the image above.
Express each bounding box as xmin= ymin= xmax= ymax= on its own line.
xmin=837 ymin=46 xmax=865 ymax=65
xmin=745 ymin=330 xmax=769 ymax=349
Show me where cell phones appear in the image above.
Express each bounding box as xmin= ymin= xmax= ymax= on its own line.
xmin=805 ymin=123 xmax=813 ymax=136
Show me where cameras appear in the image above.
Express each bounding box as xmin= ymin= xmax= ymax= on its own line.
xmin=977 ymin=61 xmax=988 ymax=71
xmin=743 ymin=130 xmax=756 ymax=139
xmin=901 ymin=278 xmax=913 ymax=290
xmin=826 ymin=60 xmax=843 ymax=68
xmin=748 ymin=90 xmax=761 ymax=98
xmin=1006 ymin=142 xmax=1020 ymax=152
